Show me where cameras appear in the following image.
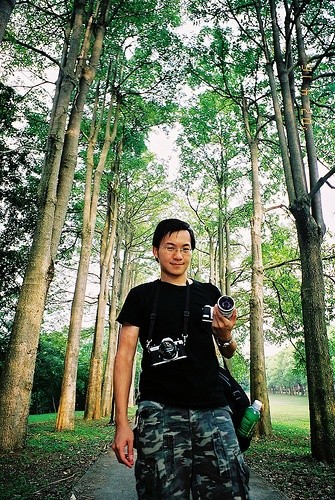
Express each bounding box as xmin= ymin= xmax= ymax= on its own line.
xmin=202 ymin=295 xmax=235 ymax=322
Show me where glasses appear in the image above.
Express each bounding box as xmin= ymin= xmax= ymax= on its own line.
xmin=159 ymin=244 xmax=191 ymax=256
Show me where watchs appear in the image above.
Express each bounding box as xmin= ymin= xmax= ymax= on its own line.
xmin=215 ymin=335 xmax=233 ymax=348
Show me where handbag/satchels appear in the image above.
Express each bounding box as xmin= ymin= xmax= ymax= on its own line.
xmin=219 ymin=367 xmax=251 ymax=453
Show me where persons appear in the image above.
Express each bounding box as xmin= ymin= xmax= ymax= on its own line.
xmin=111 ymin=218 xmax=252 ymax=499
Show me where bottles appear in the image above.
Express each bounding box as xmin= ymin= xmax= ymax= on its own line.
xmin=239 ymin=399 xmax=262 ymax=438
